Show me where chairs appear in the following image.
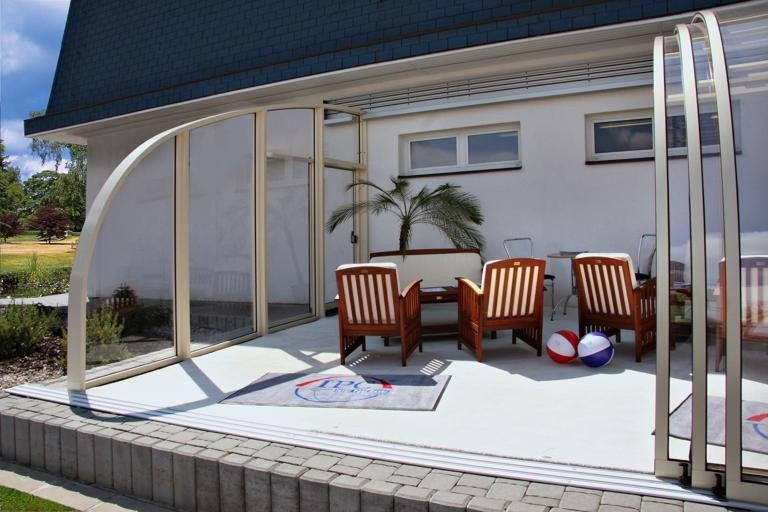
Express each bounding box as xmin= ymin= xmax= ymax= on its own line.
xmin=713 ymin=252 xmax=768 ymax=372
xmin=571 ymin=252 xmax=678 ymax=362
xmin=454 ymin=256 xmax=546 ymax=361
xmin=502 ymin=236 xmax=557 ymax=315
xmin=334 ymin=261 xmax=423 ymax=369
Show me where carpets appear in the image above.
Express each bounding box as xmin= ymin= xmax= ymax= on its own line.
xmin=650 ymin=389 xmax=768 ymax=456
xmin=219 ymin=369 xmax=452 ymax=413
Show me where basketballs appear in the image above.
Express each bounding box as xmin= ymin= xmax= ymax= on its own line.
xmin=546 ymin=330 xmax=579 ymax=363
xmin=577 ymin=332 xmax=615 ymax=368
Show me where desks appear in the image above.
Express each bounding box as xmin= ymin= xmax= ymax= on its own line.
xmin=546 ymin=251 xmax=586 ymax=321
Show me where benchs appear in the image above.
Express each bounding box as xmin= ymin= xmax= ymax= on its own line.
xmin=337 ymin=247 xmax=499 ymax=349
